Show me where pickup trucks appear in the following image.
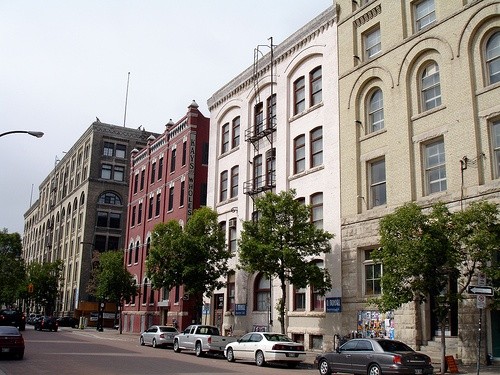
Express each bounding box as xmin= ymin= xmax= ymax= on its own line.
xmin=173 ymin=325 xmax=235 ymax=357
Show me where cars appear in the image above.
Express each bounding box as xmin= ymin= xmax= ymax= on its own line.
xmin=314 ymin=338 xmax=434 ymax=375
xmin=57 ymin=316 xmax=79 ymax=329
xmin=34 ymin=316 xmax=59 ymax=332
xmin=140 ymin=326 xmax=180 ymax=348
xmin=0 ymin=303 xmax=42 ymax=331
xmin=0 ymin=326 xmax=25 ymax=360
xmin=224 ymin=331 xmax=306 ymax=367
